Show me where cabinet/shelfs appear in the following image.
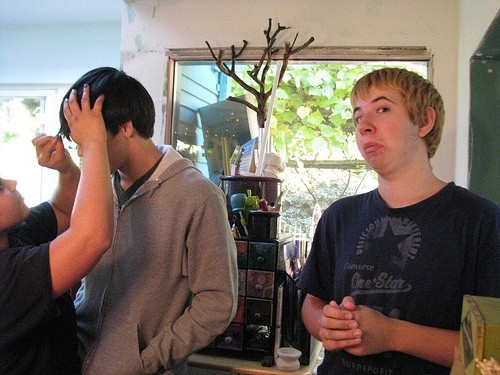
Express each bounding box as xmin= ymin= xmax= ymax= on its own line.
xmin=195 ymin=234 xmax=295 ymax=363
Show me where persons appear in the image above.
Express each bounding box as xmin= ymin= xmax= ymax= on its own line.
xmin=0 ymin=83 xmax=115 ymax=375
xmin=296 ymin=68 xmax=500 ymax=375
xmin=58 ymin=67 xmax=239 ymax=375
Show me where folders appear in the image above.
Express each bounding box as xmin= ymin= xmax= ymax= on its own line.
xmin=273 ymin=280 xmax=285 ymax=362
